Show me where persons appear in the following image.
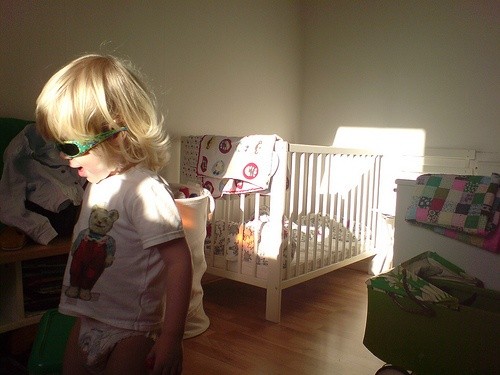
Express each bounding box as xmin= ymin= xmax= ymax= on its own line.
xmin=35 ymin=54 xmax=192 ymax=375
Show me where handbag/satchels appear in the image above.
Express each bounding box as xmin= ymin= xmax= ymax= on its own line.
xmin=173 ymin=182 xmax=216 ymax=339
xmin=365 ymin=251 xmax=483 ymax=316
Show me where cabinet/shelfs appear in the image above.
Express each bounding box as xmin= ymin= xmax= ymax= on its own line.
xmin=0 ymin=234 xmax=74 ymax=334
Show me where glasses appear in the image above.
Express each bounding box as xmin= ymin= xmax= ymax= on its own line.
xmin=55 ymin=127 xmax=127 ymax=158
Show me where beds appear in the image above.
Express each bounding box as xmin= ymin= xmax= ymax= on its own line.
xmin=179 ymin=132 xmax=384 ymax=323
